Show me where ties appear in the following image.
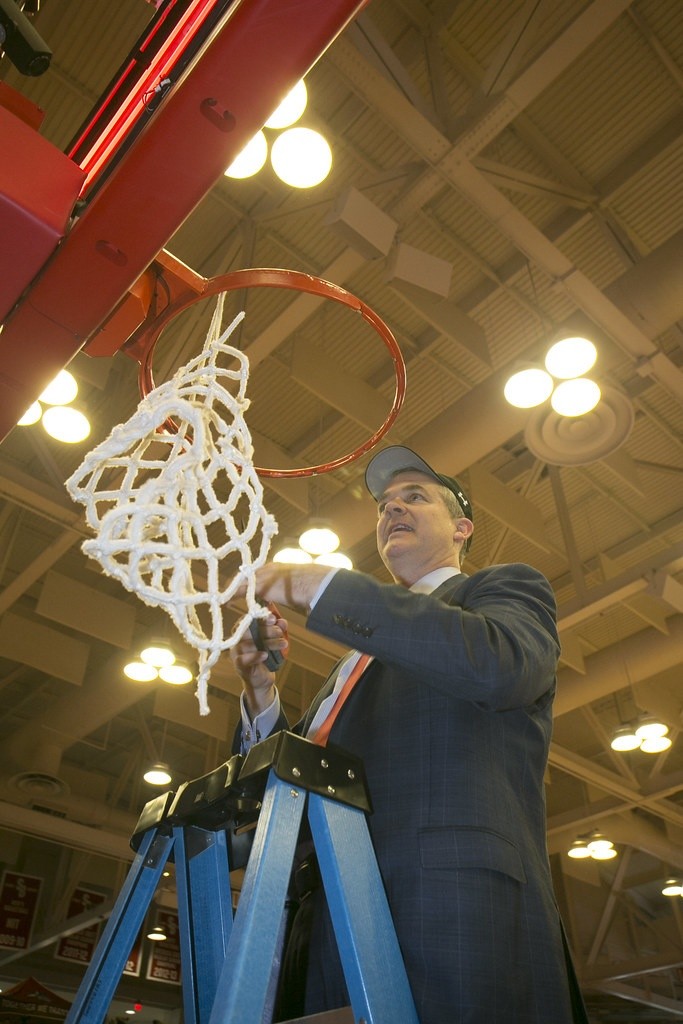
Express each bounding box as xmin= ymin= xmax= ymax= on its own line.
xmin=313 ymin=655 xmax=371 ymax=748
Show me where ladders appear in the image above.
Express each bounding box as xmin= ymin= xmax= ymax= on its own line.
xmin=65 ymin=728 xmax=421 ymax=1024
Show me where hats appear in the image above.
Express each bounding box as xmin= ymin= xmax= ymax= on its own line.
xmin=364 ymin=445 xmax=473 ymax=552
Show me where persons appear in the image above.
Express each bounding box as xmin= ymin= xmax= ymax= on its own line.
xmin=233 ymin=444 xmax=586 ymax=1024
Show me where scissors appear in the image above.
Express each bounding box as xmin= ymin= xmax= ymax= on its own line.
xmin=239 ymin=517 xmax=290 ymax=671
